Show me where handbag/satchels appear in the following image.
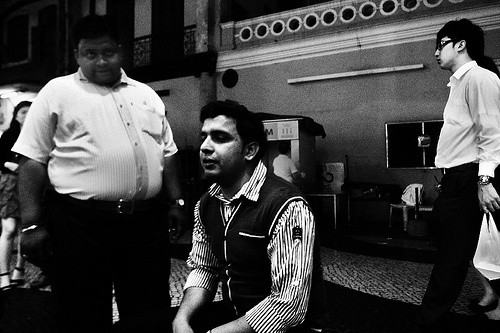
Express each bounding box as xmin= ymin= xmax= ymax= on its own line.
xmin=472 ymin=210 xmax=500 ymax=281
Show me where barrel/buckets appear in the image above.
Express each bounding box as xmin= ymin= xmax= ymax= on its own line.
xmin=407 ymin=220 xmax=429 ymax=236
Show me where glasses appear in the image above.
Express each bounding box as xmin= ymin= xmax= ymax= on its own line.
xmin=436 ymin=39 xmax=461 ymax=50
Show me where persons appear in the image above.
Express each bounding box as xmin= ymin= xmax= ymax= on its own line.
xmin=415 ymin=19 xmax=500 ymax=333
xmin=111 ymin=100 xmax=318 ymax=333
xmin=0 ymin=100 xmax=34 ymax=292
xmin=10 ymin=14 xmax=189 ymax=333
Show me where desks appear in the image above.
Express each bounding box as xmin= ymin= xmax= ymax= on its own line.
xmin=310 ymin=193 xmax=350 ymax=231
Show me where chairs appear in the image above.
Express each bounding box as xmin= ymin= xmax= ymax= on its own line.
xmin=389 ymin=183 xmax=424 ymax=231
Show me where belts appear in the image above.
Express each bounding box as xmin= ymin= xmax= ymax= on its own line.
xmin=441 ymin=162 xmax=480 ymax=176
xmin=61 ymin=193 xmax=166 ymax=215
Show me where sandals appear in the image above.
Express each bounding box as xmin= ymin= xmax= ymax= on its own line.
xmin=0 ymin=272 xmax=13 ymax=296
xmin=11 ymin=265 xmax=26 ymax=288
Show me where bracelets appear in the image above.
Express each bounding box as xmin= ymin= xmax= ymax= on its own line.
xmin=210 ymin=330 xmax=211 ymax=333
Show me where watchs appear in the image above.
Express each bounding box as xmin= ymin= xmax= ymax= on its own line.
xmin=169 ymin=198 xmax=185 ymax=206
xmin=477 ymin=175 xmax=491 ymax=185
